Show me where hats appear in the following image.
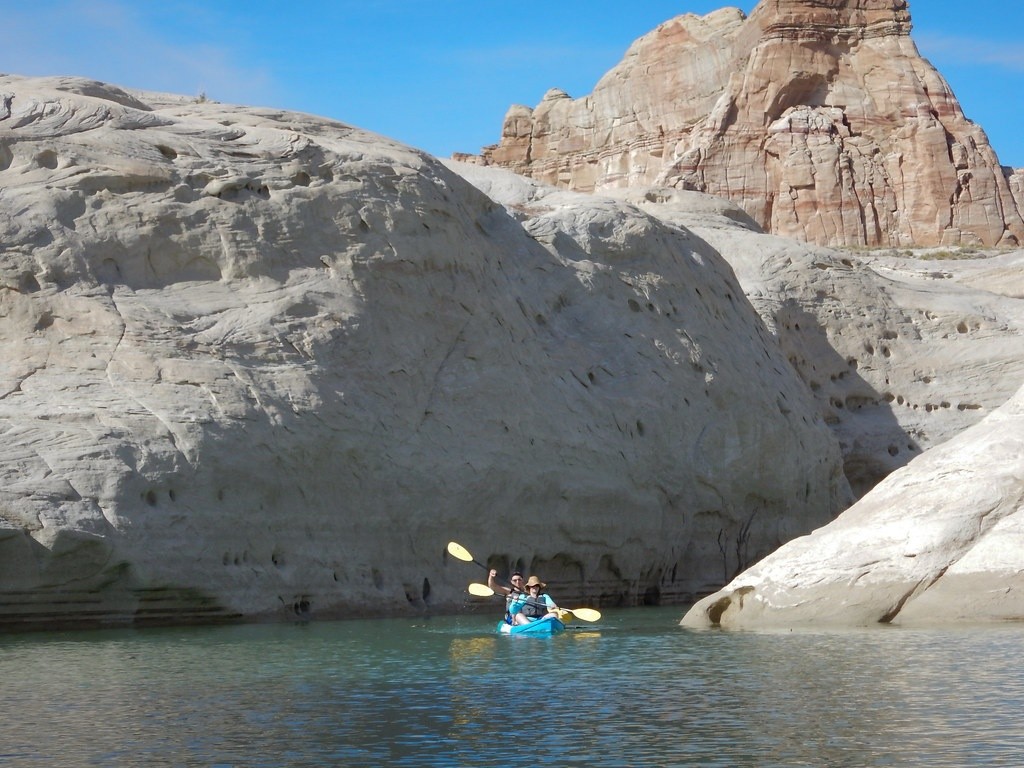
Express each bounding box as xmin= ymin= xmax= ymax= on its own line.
xmin=525 ymin=576 xmax=546 ymax=591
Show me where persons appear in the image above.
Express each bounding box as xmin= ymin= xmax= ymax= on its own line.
xmin=487 ymin=569 xmax=529 ymax=624
xmin=509 ymin=576 xmax=557 ymax=625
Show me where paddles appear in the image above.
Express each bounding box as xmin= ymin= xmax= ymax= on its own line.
xmin=447 ymin=541 xmax=572 ymax=622
xmin=468 ymin=582 xmax=602 ymax=623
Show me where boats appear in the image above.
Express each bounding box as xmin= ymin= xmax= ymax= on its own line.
xmin=496 ymin=617 xmax=565 ymax=634
